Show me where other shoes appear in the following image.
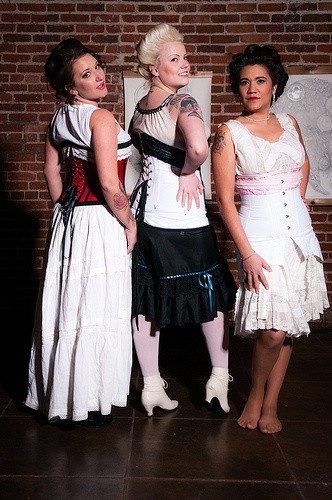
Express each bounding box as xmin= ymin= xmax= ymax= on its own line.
xmin=77 ymin=413 xmax=113 ymax=427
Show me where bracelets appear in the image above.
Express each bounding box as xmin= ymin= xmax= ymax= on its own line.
xmin=241 ymin=250 xmax=255 ymax=261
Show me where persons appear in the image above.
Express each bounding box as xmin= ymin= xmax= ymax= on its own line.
xmin=212 ymin=44 xmax=331 ymax=433
xmin=128 ymin=25 xmax=239 ymax=416
xmin=21 ymin=39 xmax=136 ymax=426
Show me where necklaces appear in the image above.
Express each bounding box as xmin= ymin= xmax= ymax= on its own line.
xmin=242 ymin=112 xmax=270 ymax=122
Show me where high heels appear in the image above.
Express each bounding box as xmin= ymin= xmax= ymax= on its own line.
xmin=141 ymin=372 xmax=179 ymax=416
xmin=205 ymin=369 xmax=231 ymax=414
